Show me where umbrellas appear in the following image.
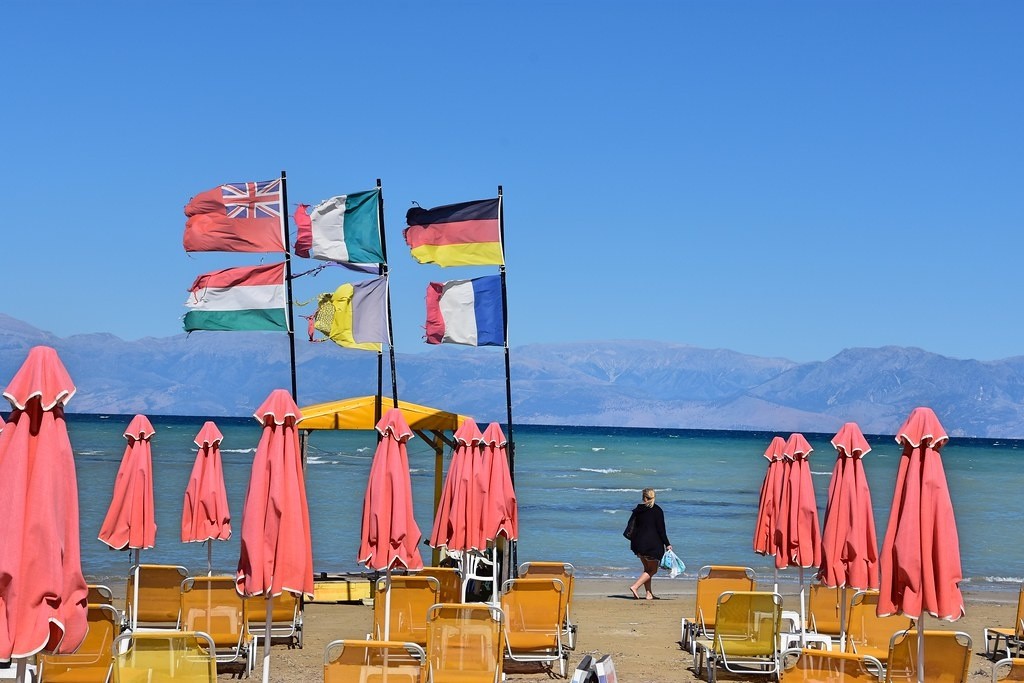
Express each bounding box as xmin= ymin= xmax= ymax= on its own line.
xmin=817 ymin=422 xmax=880 ymax=683
xmin=429 ymin=419 xmax=482 ymax=672
xmin=783 ymin=433 xmax=823 ymax=679
xmin=355 ymin=410 xmax=422 ymax=683
xmin=182 ymin=421 xmax=236 ymax=636
xmin=482 ymin=422 xmax=518 ymax=635
xmin=236 ymin=390 xmax=314 ymax=683
xmin=753 ymin=437 xmax=790 ymax=635
xmin=0 ymin=345 xmax=88 ymax=683
xmin=98 ymin=415 xmax=160 ymax=668
xmin=880 ymin=408 xmax=967 ymax=683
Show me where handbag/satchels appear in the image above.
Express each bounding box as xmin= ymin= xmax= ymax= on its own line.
xmin=659 ymin=549 xmax=686 ymax=579
xmin=623 ymin=514 xmax=636 ymax=539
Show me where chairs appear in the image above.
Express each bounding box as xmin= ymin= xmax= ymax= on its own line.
xmin=37 ymin=564 xmax=304 ymax=683
xmin=984 ymin=581 xmax=1024 ymax=683
xmin=323 ymin=561 xmax=578 ymax=683
xmin=681 ymin=565 xmax=973 ymax=683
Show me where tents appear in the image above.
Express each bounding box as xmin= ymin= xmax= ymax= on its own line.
xmin=297 ymin=396 xmax=474 ymax=567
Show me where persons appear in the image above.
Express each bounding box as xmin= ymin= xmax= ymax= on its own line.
xmin=623 ymin=488 xmax=673 ymax=600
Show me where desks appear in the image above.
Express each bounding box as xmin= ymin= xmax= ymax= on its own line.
xmin=186 ymin=605 xmax=239 ymax=651
xmin=440 ymin=625 xmax=494 ymax=671
xmin=465 ymin=602 xmax=510 ymax=634
xmin=777 ymin=633 xmax=832 ymax=677
xmin=0 ymin=663 xmax=38 ymax=683
xmin=753 ymin=610 xmax=800 ymax=648
xmin=118 ymin=628 xmax=182 ymax=677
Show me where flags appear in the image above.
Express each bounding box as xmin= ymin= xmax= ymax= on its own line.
xmin=419 ymin=274 xmax=504 ymax=346
xmin=298 ymin=275 xmax=393 ymax=343
xmin=294 ymin=283 xmax=384 ymax=353
xmin=292 ymin=263 xmax=379 ymax=278
xmin=292 ymin=188 xmax=386 ymax=263
xmin=401 ymin=196 xmax=506 ymax=267
xmin=184 ymin=178 xmax=290 ymax=253
xmin=181 ymin=262 xmax=291 ymax=332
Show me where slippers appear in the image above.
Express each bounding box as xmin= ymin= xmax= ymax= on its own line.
xmin=646 ymin=596 xmax=660 ymax=600
xmin=630 ymin=588 xmax=639 ymax=599
